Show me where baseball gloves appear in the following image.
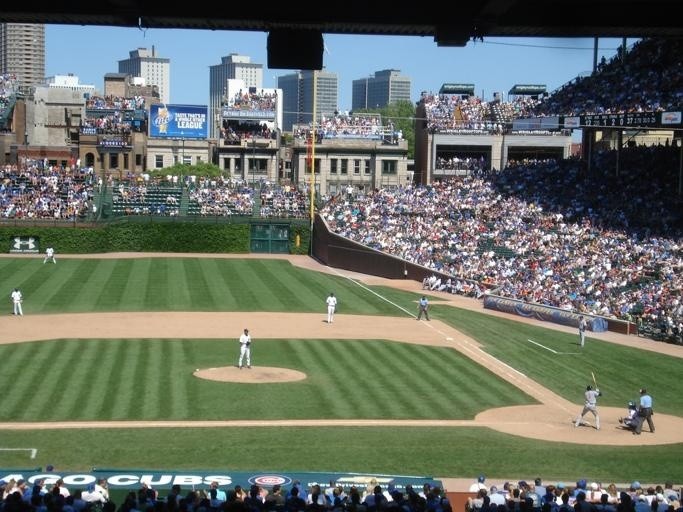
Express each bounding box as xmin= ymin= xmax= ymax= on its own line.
xmin=619 ymin=417 xmax=623 ymax=423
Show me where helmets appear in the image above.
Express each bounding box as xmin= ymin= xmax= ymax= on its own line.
xmin=640 ymin=388 xmax=646 ymax=394
xmin=628 ymin=401 xmax=636 ymax=409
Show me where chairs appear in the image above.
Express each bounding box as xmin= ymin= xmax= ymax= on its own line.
xmin=0 ymin=32 xmax=682 ymax=342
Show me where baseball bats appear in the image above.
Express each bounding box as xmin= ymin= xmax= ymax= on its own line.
xmin=590 ymin=372 xmax=599 ymax=390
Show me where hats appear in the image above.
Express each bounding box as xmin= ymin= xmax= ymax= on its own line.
xmin=474 ymin=475 xmax=680 ymax=503
xmin=586 ymin=384 xmax=593 ymax=390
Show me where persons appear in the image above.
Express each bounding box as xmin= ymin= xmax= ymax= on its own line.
xmin=634 ymin=387 xmax=656 ymax=434
xmin=618 ymin=400 xmax=639 ymax=428
xmin=574 ymin=385 xmax=602 ymax=430
xmin=238 ymin=329 xmax=251 ymax=369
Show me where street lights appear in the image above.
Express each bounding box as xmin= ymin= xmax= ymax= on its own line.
xmin=181 ymin=136 xmax=186 ymax=165
xmin=251 ymin=136 xmax=256 ymax=190
xmin=24 ymin=132 xmax=29 ymax=169
